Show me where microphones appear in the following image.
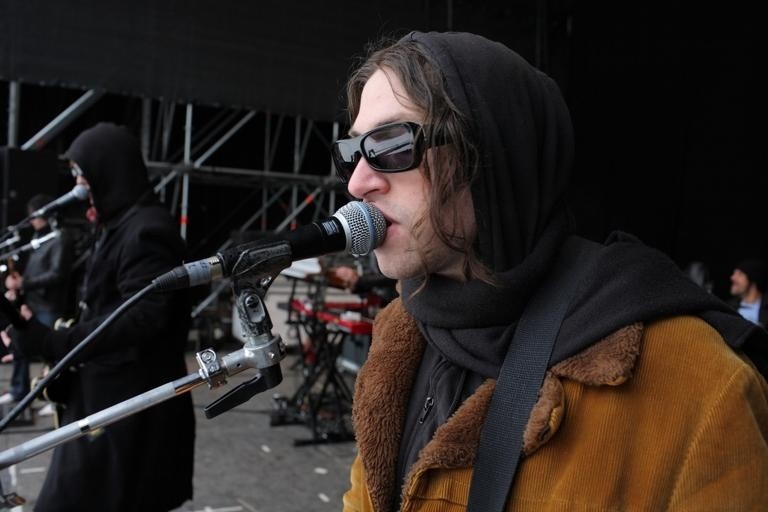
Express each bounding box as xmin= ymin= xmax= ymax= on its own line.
xmin=31 ymin=184 xmax=88 ymax=218
xmin=155 ymin=201 xmax=387 ymax=289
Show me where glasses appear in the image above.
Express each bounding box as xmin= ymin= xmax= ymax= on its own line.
xmin=331 ymin=122 xmax=450 ymax=186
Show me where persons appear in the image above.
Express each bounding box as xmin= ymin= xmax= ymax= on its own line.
xmin=333 ymin=252 xmax=389 ymax=293
xmin=1 ymin=119 xmax=197 ymax=510
xmin=728 ymin=257 xmax=764 ymax=327
xmin=330 ymin=30 xmax=764 ymax=509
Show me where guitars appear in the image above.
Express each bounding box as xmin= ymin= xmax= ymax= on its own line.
xmin=3 ymin=289 xmax=75 ymax=429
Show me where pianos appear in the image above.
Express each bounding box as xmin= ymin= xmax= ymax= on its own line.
xmin=284 ymin=287 xmax=373 ymax=335
